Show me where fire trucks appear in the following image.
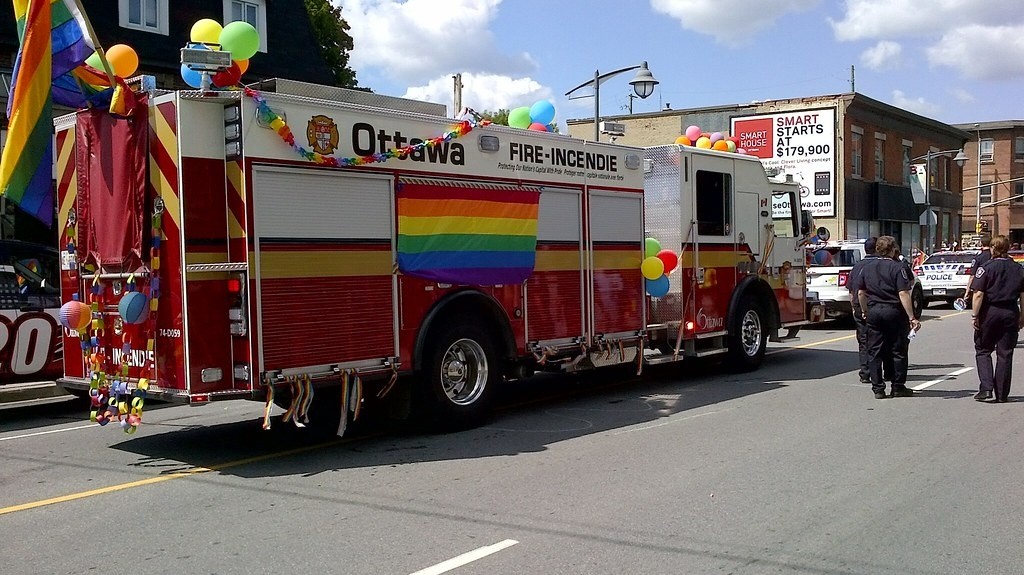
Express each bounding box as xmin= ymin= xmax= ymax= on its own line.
xmin=50 ymin=75 xmax=810 ymax=431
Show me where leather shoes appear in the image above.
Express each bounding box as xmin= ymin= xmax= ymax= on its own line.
xmin=860 ymin=377 xmax=871 ymax=383
xmin=974 ymin=390 xmax=1007 ymax=403
xmin=874 ymin=384 xmax=913 ymax=399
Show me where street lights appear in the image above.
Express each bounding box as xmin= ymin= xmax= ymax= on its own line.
xmin=565 ymin=59 xmax=662 ymax=142
xmin=910 ymin=148 xmax=970 ymax=258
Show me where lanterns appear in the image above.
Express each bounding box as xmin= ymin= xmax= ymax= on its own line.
xmin=59 ymin=301 xmax=93 ymax=331
xmin=117 ymin=291 xmax=151 ymax=324
xmin=814 ymin=250 xmax=832 ymax=265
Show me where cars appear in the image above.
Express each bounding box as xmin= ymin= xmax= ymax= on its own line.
xmin=913 ymin=250 xmax=981 ymax=308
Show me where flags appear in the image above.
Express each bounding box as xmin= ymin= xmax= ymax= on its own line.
xmin=0 ymin=0 xmax=138 ymax=228
xmin=908 ymin=163 xmax=927 ymax=204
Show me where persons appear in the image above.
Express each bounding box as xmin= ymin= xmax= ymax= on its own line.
xmin=964 ymin=235 xmax=1024 ymax=403
xmin=846 ymin=236 xmax=921 ymax=399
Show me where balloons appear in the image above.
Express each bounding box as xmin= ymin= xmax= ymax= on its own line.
xmin=106 ymin=44 xmax=139 ymax=78
xmin=736 ymin=148 xmax=746 ymax=155
xmin=675 ymin=134 xmax=691 ymax=147
xmin=546 ymin=125 xmax=553 ymax=132
xmin=686 ymin=125 xmax=739 ymax=154
xmin=507 ymin=106 xmax=532 ymax=130
xmin=233 ymin=60 xmax=249 ymax=74
xmin=529 ymin=100 xmax=556 ymax=126
xmin=82 ymin=55 xmax=115 ymax=91
xmin=190 ymin=19 xmax=223 ymax=52
xmin=645 ymin=238 xmax=661 ymax=257
xmin=656 ymin=249 xmax=678 ymax=272
xmin=218 ymin=21 xmax=260 ymax=61
xmin=180 ymin=45 xmax=213 ymax=88
xmin=645 ymin=272 xmax=671 ymax=297
xmin=210 ymin=58 xmax=242 ymax=90
xmin=641 ymin=255 xmax=664 ymax=280
xmin=527 ymin=123 xmax=548 ymax=133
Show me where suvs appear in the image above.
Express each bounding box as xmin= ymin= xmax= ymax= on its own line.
xmin=808 ymin=240 xmax=923 ymax=320
